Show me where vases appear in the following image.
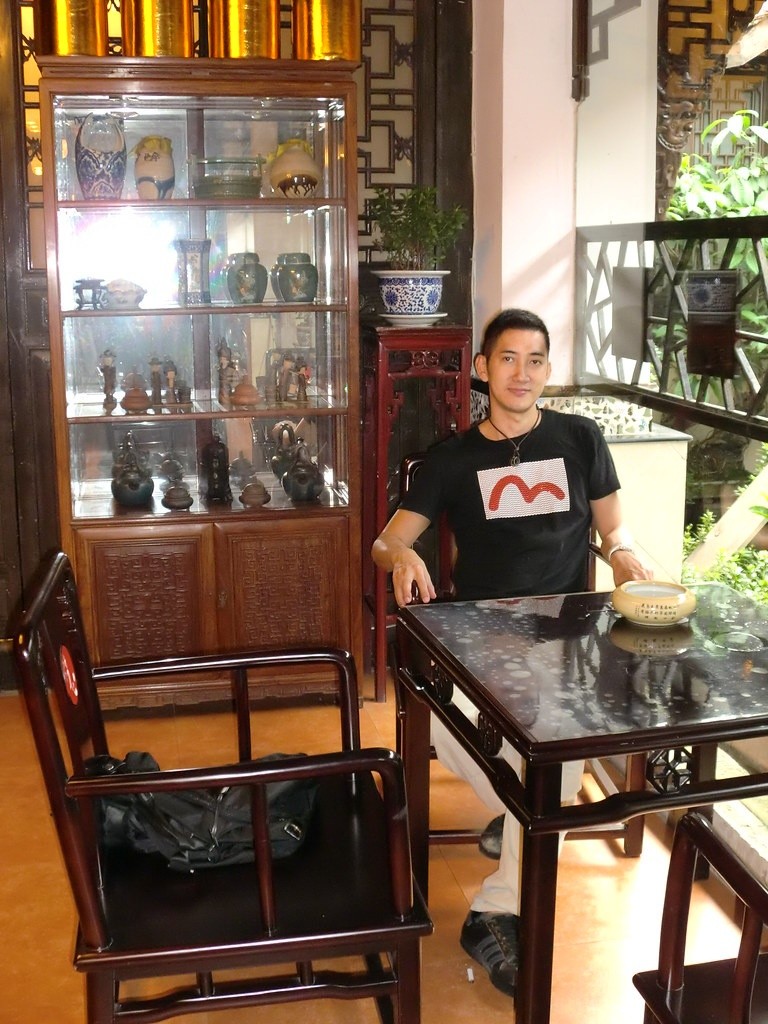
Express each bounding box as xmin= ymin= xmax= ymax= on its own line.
xmin=686 ymin=270 xmax=735 ymax=312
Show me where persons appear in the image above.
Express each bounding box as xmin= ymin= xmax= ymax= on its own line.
xmin=371 ymin=308 xmax=655 ymax=998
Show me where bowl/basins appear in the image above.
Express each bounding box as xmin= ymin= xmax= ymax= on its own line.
xmin=611 ymin=579 xmax=698 ymax=628
xmin=610 ymin=618 xmax=695 ymax=658
xmin=95 ymin=287 xmax=147 ymax=308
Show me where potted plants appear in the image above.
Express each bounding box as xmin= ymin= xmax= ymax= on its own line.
xmin=358 ymin=183 xmax=469 ymax=315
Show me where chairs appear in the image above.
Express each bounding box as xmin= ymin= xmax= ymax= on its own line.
xmin=631 ymin=811 xmax=768 ymax=1024
xmin=401 ymin=449 xmax=646 ymax=858
xmin=12 ymin=552 xmax=434 ymax=1024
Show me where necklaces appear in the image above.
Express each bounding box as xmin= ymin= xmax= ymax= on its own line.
xmin=488 ymin=406 xmax=540 ymax=466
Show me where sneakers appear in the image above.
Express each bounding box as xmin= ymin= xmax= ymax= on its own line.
xmin=460 ymin=911 xmax=519 ymax=998
xmin=480 ymin=813 xmax=505 ymax=859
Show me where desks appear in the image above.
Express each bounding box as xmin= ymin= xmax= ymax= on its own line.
xmin=362 ymin=319 xmax=471 ymax=703
xmin=395 ymin=582 xmax=768 ymax=1024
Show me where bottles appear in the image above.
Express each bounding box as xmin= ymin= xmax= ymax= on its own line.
xmin=111 ymin=432 xmax=154 ymax=506
xmin=276 ymin=253 xmax=319 ymax=303
xmin=202 ymin=434 xmax=232 ymax=504
xmin=269 ymin=253 xmax=283 ymax=303
xmin=134 ymin=135 xmax=175 ymax=200
xmin=220 ymin=254 xmax=239 ymax=302
xmin=270 ymin=140 xmax=320 ymax=199
xmin=226 ymin=252 xmax=267 ymax=304
xmin=281 ymin=437 xmax=325 ymax=502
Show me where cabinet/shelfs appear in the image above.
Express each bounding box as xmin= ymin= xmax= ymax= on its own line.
xmin=36 ymin=54 xmax=365 ymax=712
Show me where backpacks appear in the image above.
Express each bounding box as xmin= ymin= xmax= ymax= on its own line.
xmin=84 ymin=751 xmax=312 ymax=868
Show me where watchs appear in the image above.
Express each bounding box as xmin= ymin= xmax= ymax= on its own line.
xmin=607 ymin=542 xmax=636 ymax=562
